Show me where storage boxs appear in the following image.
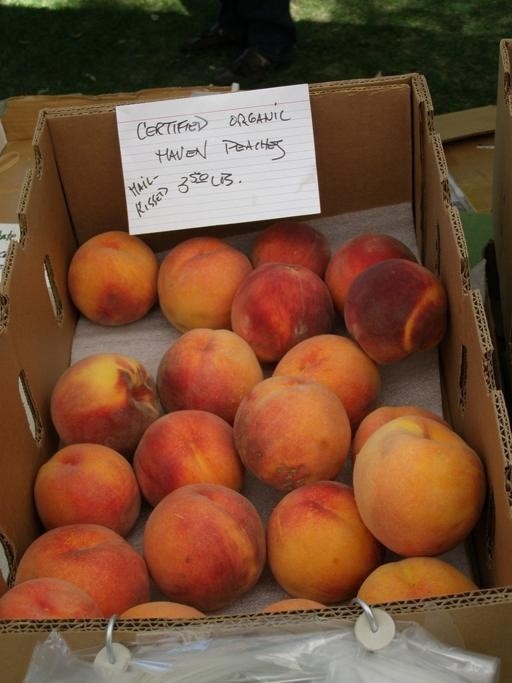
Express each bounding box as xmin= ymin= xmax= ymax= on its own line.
xmin=1 ymin=84 xmax=236 ymax=286
xmin=493 ymin=39 xmax=512 ymax=351
xmin=1 ymin=74 xmax=512 ymax=683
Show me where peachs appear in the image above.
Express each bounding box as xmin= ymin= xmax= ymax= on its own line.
xmin=1 ymin=221 xmax=487 ymax=622
